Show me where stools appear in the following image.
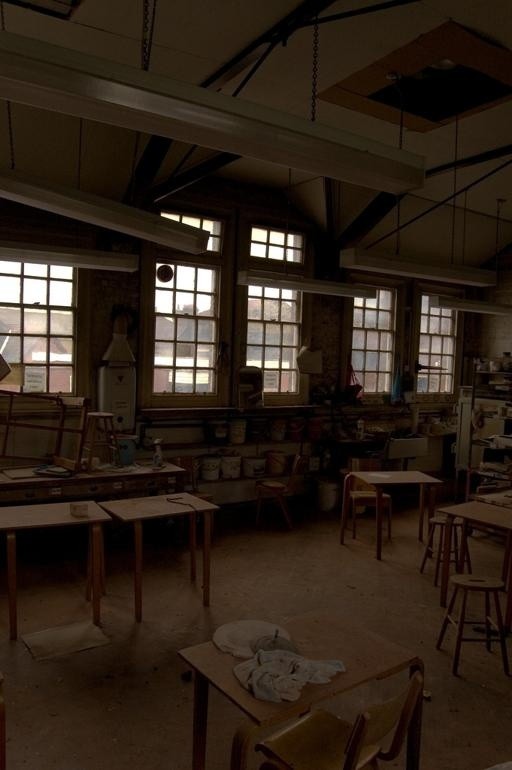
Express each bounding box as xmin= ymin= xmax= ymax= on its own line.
xmin=436 ymin=573 xmax=511 ymax=678
xmin=79 ymin=412 xmax=122 ymax=474
xmin=420 ymin=519 xmax=471 ymax=584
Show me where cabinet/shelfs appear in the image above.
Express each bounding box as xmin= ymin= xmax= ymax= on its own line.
xmin=140 ymin=405 xmax=441 ymax=484
xmin=455 ymin=384 xmax=511 ymax=501
xmin=470 ymin=359 xmax=511 ymax=494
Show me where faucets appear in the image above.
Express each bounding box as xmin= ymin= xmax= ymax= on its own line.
xmin=400 ymin=406 xmax=411 ymax=416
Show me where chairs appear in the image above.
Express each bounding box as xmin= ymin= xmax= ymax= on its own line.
xmin=253 ymin=672 xmax=424 ymax=770
xmin=349 ymin=458 xmax=392 ymax=541
xmin=256 ymin=455 xmax=304 ymax=530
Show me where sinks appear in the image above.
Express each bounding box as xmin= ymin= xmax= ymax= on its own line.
xmin=385 ymin=433 xmax=429 ymax=459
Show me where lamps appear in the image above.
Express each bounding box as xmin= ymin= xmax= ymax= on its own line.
xmin=2 ymin=203 xmax=143 ymax=277
xmin=424 ymin=119 xmax=512 ymax=316
xmin=2 ymin=116 xmax=214 ymax=257
xmin=235 ymin=155 xmax=380 ymax=302
xmin=337 ymin=99 xmax=504 ymax=292
xmin=3 ymin=3 xmax=433 ymax=201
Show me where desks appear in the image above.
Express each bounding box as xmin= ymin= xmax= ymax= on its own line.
xmin=0 ymin=499 xmax=112 ymax=640
xmin=178 ymin=611 xmax=424 ymax=770
xmin=96 ymin=493 xmax=223 ymax=623
xmin=436 ymin=501 xmax=512 ymax=636
xmin=339 ymin=470 xmax=444 ymax=561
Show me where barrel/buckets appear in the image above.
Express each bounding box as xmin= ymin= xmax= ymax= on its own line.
xmin=290 ymin=417 xmax=305 ymax=441
xmin=221 ymin=454 xmax=241 ymax=479
xmin=230 ymin=420 xmax=246 ymax=444
xmin=199 ymin=457 xmax=221 ymax=480
xmin=110 ymin=432 xmax=141 ymax=465
xmin=270 ymin=420 xmax=289 ymax=440
xmin=308 ymin=416 xmax=322 ymax=440
xmin=208 ymin=420 xmax=229 ymax=445
xmin=318 ymin=478 xmax=340 ymax=512
xmin=265 ymin=449 xmax=290 ymax=474
